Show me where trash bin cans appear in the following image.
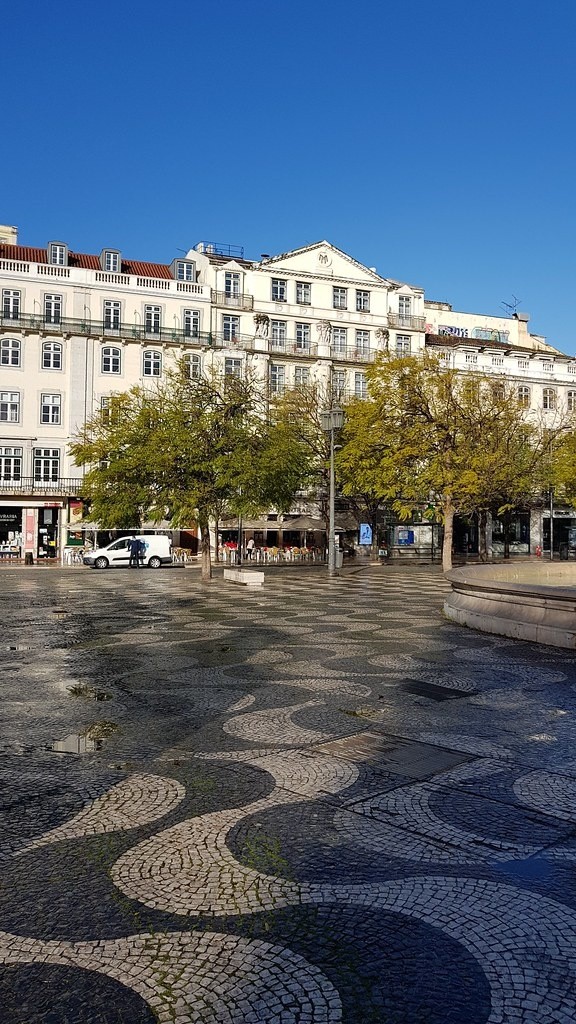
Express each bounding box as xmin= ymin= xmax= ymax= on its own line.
xmin=25 ymin=552 xmax=33 ymax=564
xmin=558 ymin=542 xmax=568 ymax=560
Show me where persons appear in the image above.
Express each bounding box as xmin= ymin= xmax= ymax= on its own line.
xmin=128 ymin=535 xmax=141 ymax=569
xmin=246 ymin=537 xmax=255 ymax=560
xmin=362 ymin=527 xmax=371 ymax=539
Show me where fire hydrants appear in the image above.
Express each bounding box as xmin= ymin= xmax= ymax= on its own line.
xmin=536 ymin=545 xmax=542 ymax=558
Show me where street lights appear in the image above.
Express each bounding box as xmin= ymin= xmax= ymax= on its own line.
xmin=318 ymin=406 xmax=346 ymax=577
xmin=550 ymin=486 xmax=555 ymax=560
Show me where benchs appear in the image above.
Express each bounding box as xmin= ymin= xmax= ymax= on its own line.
xmin=224 ymin=569 xmax=265 ymax=585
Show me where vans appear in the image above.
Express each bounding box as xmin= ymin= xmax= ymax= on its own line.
xmin=83 ymin=535 xmax=172 ymax=569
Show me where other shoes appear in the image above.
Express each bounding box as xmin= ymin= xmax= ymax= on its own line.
xmin=137 ymin=567 xmax=139 ymax=569
xmin=128 ymin=566 xmax=131 ymax=569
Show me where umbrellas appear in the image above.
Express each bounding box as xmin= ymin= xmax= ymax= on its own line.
xmin=210 ymin=515 xmax=343 ymax=547
